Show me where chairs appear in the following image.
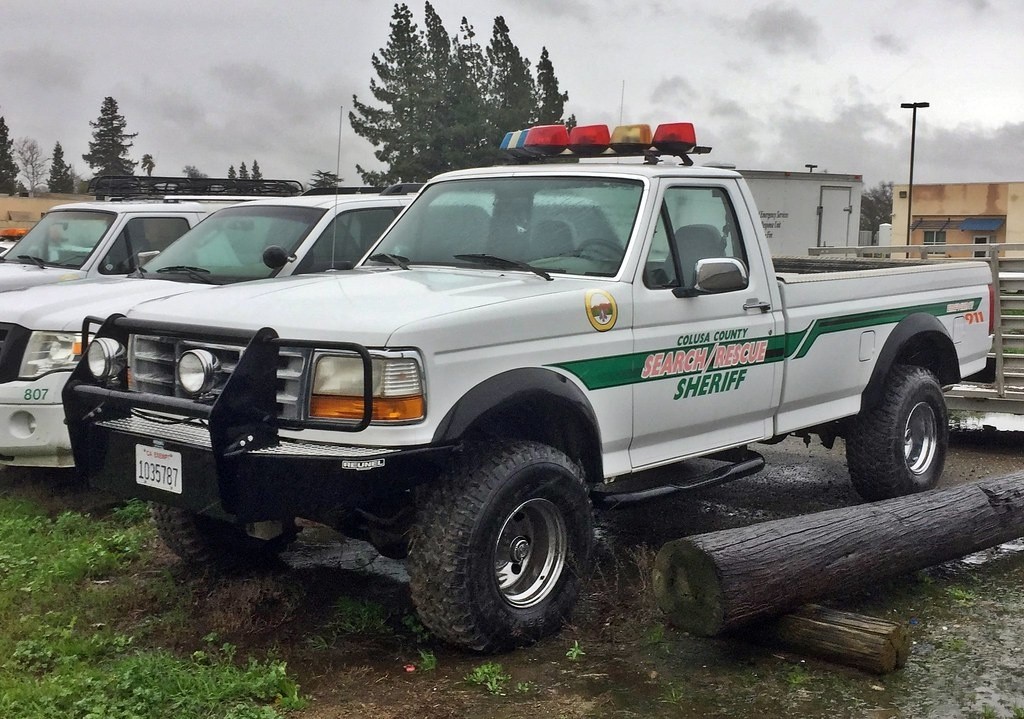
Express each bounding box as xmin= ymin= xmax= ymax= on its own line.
xmin=665 ymin=225 xmax=725 ymax=282
xmin=529 ymin=220 xmax=578 ymax=260
xmin=313 ymin=219 xmax=357 ymax=265
xmin=105 ymin=220 xmax=151 ymax=266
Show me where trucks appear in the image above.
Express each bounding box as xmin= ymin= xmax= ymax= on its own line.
xmin=562 ymin=166 xmax=865 ymax=258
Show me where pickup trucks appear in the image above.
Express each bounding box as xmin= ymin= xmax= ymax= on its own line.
xmin=62 ymin=124 xmax=993 ymax=658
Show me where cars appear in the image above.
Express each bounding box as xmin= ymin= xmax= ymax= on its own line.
xmin=0 ymin=228 xmax=92 ymax=263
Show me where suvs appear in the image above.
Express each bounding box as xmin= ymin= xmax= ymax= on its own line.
xmin=0 ymin=177 xmax=625 ymax=469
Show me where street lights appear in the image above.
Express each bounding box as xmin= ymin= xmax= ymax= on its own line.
xmin=805 ymin=165 xmax=816 ymax=172
xmin=901 ymin=101 xmax=930 ymax=260
